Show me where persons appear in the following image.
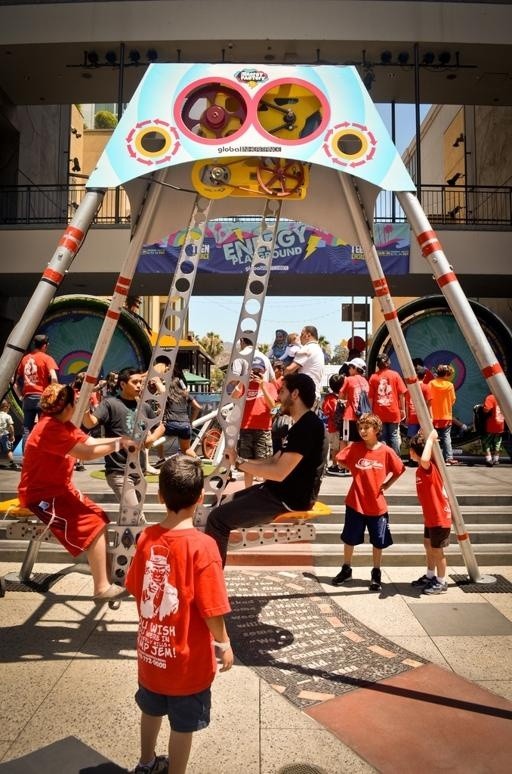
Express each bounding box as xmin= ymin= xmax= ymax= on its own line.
xmin=123 ymin=455 xmax=234 ymax=773
xmin=0 ymin=327 xmax=456 ymax=602
xmin=473 ymin=393 xmax=505 ymax=467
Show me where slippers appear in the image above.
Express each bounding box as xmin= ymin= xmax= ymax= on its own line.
xmin=88 ymin=582 xmax=127 ymax=600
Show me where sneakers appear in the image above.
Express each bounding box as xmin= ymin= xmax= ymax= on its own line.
xmin=326 ymin=465 xmax=352 ymax=477
xmin=135 ymin=754 xmax=170 ymax=774
xmin=411 ymin=575 xmax=436 ymax=588
xmin=369 ymin=568 xmax=383 ymax=593
xmin=332 ymin=565 xmax=352 ymax=586
xmin=421 ymin=576 xmax=447 ymax=595
xmin=76 ymin=463 xmax=84 ymax=471
xmin=485 ymin=460 xmax=499 ymax=466
xmin=154 ymin=458 xmax=166 ymax=468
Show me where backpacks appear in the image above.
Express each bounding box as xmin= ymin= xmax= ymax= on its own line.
xmin=352 ymin=376 xmax=372 ymax=416
xmin=326 ymin=393 xmax=345 ymax=430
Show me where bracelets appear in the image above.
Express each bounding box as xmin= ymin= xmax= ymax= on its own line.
xmin=213 ymin=638 xmax=230 ymax=652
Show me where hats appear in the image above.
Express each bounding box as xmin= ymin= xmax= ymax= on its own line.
xmin=251 ymin=357 xmax=265 ymax=371
xmin=344 ymin=358 xmax=368 ymax=373
